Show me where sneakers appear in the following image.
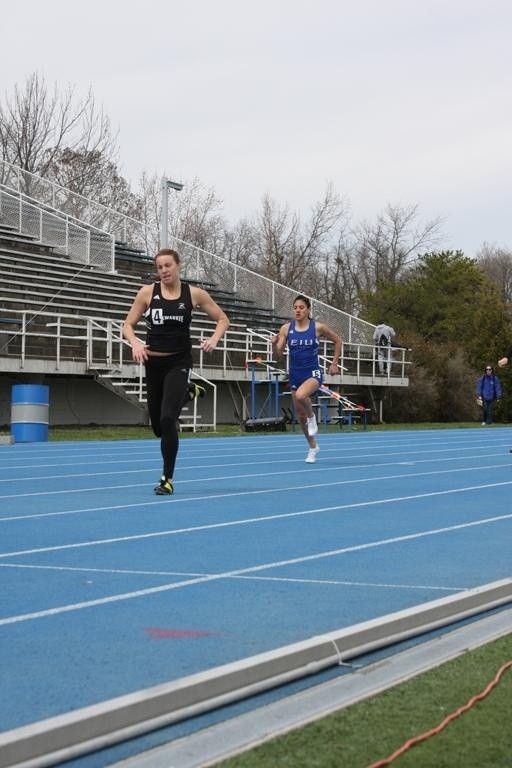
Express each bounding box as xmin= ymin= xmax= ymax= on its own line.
xmin=153 ymin=475 xmax=174 ymax=495
xmin=305 ymin=444 xmax=320 ymax=463
xmin=306 ymin=412 xmax=319 ymax=437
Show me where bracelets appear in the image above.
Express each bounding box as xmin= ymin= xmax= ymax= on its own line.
xmin=333 ymin=361 xmax=337 ymax=366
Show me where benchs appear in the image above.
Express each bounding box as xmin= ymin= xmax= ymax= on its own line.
xmin=283 ymin=390 xmax=371 ymax=434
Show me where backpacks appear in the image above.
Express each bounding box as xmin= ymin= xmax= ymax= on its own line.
xmin=378 ymin=326 xmax=390 ymax=346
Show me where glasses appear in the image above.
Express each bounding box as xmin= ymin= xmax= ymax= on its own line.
xmin=486 ymin=368 xmax=492 ymax=370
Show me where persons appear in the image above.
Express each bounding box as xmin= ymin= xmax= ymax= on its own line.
xmin=478 ymin=365 xmax=502 ymax=425
xmin=496 ymin=346 xmax=511 ymax=455
xmin=121 ymin=248 xmax=230 ymax=497
xmin=369 ymin=319 xmax=399 ymax=380
xmin=269 ymin=295 xmax=343 ymax=464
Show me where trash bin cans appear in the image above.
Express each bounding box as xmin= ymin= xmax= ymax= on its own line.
xmin=10 ymin=384 xmax=50 ymax=444
